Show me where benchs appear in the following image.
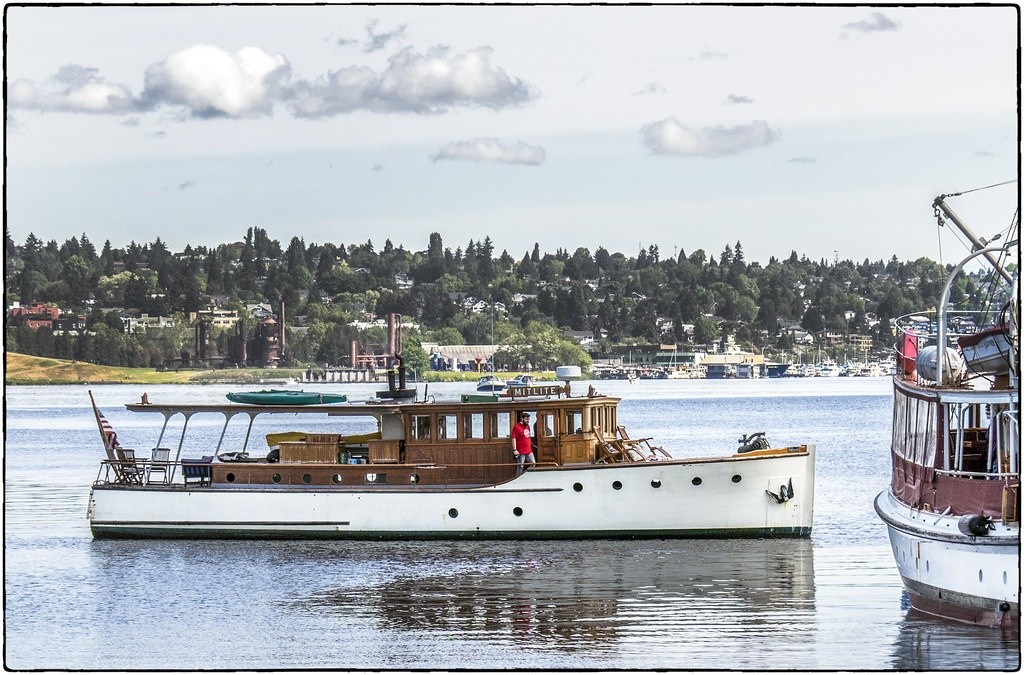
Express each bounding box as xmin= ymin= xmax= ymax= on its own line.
xmin=180 ymin=459 xmax=257 ymax=487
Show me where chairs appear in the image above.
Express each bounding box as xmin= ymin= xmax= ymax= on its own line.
xmin=116 ymin=449 xmax=144 ymax=485
xmin=146 ymin=448 xmax=170 ymax=484
xmin=616 ymin=425 xmax=671 ymax=459
xmin=592 ymin=426 xmax=647 ymax=464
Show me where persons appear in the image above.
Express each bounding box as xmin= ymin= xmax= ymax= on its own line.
xmin=534 ymin=413 xmax=552 ymax=461
xmin=511 ymin=413 xmax=537 ymax=476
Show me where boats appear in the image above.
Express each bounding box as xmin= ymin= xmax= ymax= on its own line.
xmin=873 ymin=180 xmax=1019 ymax=631
xmin=803 ymin=352 xmax=893 ymax=378
xmin=477 ymin=371 xmax=507 ymax=392
xmin=88 ymin=312 xmax=816 ymax=538
xmin=226 ymin=390 xmax=346 ymax=405
xmin=505 ymin=373 xmax=539 ymax=387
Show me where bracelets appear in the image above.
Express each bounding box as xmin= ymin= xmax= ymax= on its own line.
xmin=513 ymin=449 xmax=518 ymax=451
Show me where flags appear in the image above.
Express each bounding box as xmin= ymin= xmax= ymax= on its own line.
xmin=95 ymin=406 xmax=119 ymax=449
